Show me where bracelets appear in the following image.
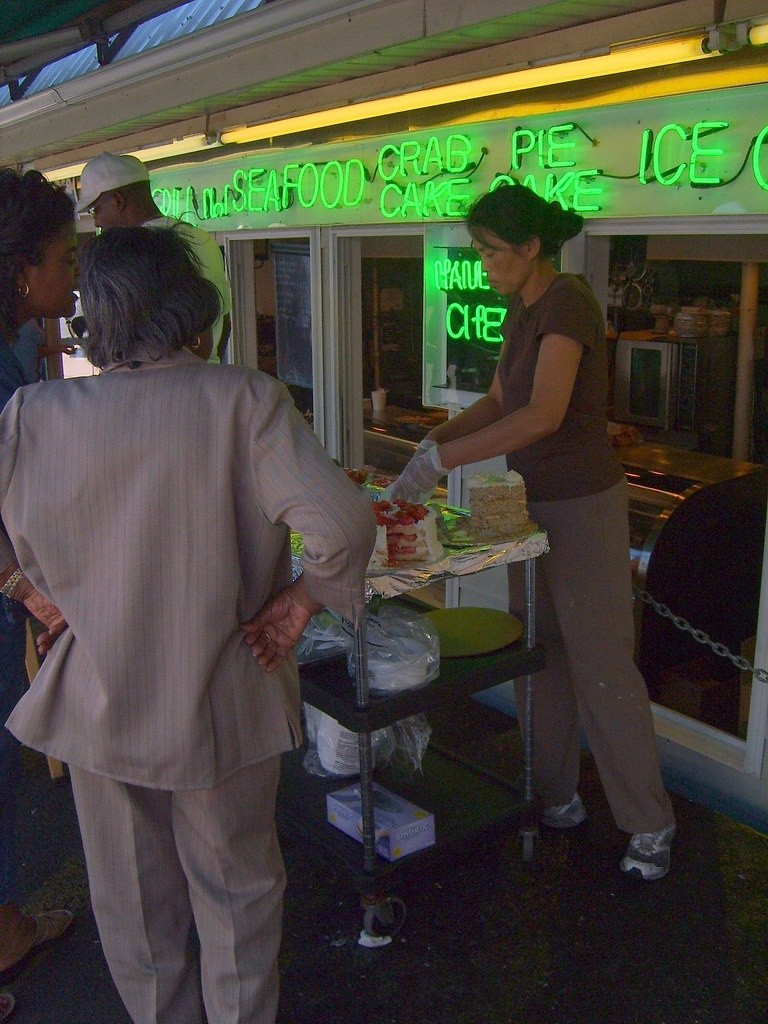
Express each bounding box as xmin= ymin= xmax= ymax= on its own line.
xmin=0 ymin=567 xmax=22 ymax=597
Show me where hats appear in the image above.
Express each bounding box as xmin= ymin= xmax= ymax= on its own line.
xmin=74 ymin=151 xmax=149 ymax=214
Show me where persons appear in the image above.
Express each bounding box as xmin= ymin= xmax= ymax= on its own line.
xmin=1 ymin=223 xmax=378 ymax=1024
xmin=0 ymin=165 xmax=84 ymax=1024
xmin=389 ymin=184 xmax=677 ymax=881
xmin=75 ymin=153 xmax=239 ymax=365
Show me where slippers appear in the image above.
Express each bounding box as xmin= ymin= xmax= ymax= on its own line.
xmin=0 ymin=909 xmax=74 ymax=984
xmin=0 ymin=995 xmax=15 ymax=1022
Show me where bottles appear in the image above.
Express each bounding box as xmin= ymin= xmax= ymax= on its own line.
xmin=674 ymin=312 xmax=708 ymax=338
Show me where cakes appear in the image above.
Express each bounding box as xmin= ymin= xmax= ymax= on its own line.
xmin=373 ymin=498 xmax=442 ymax=565
xmin=467 ymin=469 xmax=529 ymax=529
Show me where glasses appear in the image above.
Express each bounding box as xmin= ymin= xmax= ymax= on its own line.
xmin=87 ymin=191 xmax=112 ymax=217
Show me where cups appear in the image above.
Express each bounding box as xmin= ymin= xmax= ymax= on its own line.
xmin=372 ymin=391 xmax=386 ymax=411
xmin=705 ymin=310 xmax=733 ymax=337
xmin=650 ymin=315 xmax=669 ymax=334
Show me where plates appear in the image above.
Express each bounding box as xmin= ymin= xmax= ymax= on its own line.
xmin=438 ymin=518 xmax=538 ymax=546
xmin=422 ymin=607 xmax=524 ymax=659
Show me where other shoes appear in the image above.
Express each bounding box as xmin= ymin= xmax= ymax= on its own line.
xmin=4 ymin=597 xmax=27 ymax=626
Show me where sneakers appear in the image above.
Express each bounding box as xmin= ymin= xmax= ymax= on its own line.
xmin=543 ymin=793 xmax=588 ymax=829
xmin=621 ymin=828 xmax=677 ymax=881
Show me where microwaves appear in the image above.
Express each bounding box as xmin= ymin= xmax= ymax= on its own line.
xmin=610 ymin=331 xmax=738 ymax=433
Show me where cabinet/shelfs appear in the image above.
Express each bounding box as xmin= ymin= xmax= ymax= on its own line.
xmin=266 ymin=486 xmax=551 ymax=947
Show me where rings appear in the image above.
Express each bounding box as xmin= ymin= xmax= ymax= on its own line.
xmin=267 ymin=632 xmax=271 ymax=642
